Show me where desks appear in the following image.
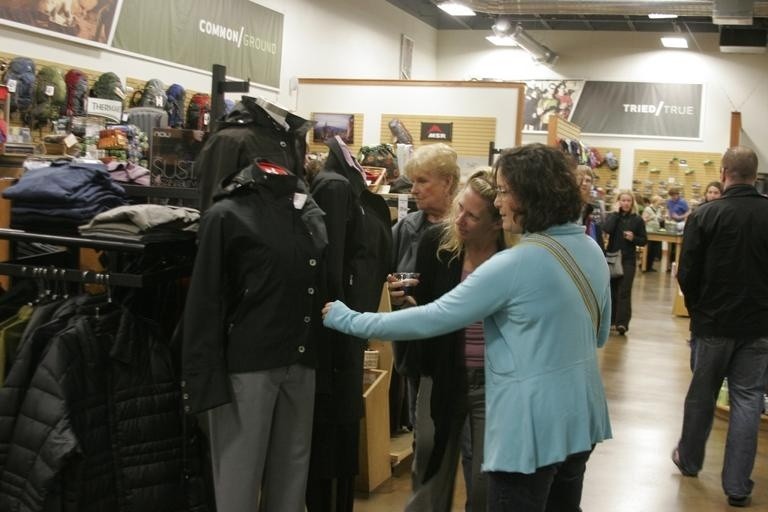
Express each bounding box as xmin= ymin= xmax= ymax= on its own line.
xmin=641 ymin=232 xmax=684 ymax=273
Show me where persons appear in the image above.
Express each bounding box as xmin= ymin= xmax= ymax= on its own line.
xmin=177 ymin=152 xmax=335 ymax=511
xmin=187 ymin=92 xmax=319 ymax=221
xmin=382 ymin=161 xmax=528 ymax=512
xmin=317 ymin=142 xmax=619 ymax=512
xmin=573 ymin=164 xmax=727 ymax=339
xmin=668 ymin=143 xmax=767 ymax=508
xmin=377 ymin=140 xmax=464 ymax=452
xmin=302 ymin=134 xmax=399 ymax=512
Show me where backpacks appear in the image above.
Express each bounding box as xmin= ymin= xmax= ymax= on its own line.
xmin=137 ymin=78 xmax=236 ymax=132
xmin=91 ymin=71 xmax=126 ymax=112
xmin=28 ymin=64 xmax=68 ymax=121
xmin=2 ymin=56 xmax=36 ymax=114
xmin=63 ymin=67 xmax=89 ymax=117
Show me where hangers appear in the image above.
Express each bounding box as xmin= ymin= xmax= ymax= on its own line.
xmin=21 ymin=265 xmax=113 ymax=318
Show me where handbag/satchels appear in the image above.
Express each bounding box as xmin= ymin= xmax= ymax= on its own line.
xmin=604 ymin=248 xmax=625 ymax=279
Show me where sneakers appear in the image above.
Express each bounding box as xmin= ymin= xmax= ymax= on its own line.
xmin=728 ymin=495 xmax=749 ymax=506
xmin=610 ymin=324 xmax=626 ymax=335
xmin=673 ymin=448 xmax=698 ymax=477
